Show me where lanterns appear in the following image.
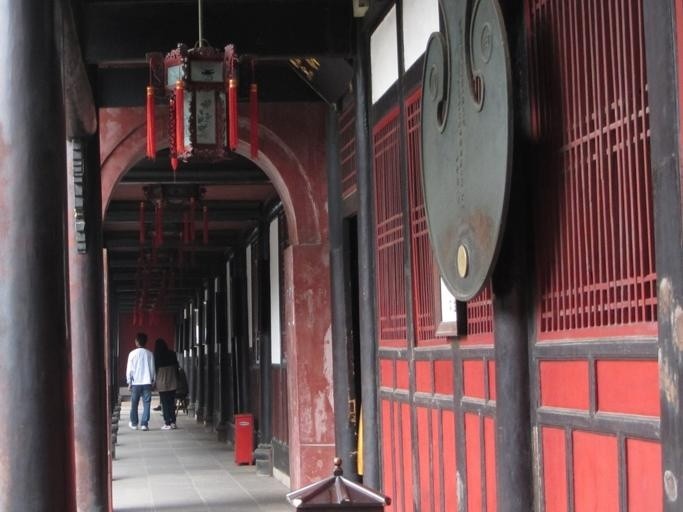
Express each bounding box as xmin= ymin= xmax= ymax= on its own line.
xmin=129 ymin=180 xmax=211 ymax=332
xmin=142 ymin=43 xmax=264 ymax=172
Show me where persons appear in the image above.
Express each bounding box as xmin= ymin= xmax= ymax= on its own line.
xmin=125 ymin=333 xmax=155 ymax=431
xmin=154 ymin=337 xmax=180 ymax=429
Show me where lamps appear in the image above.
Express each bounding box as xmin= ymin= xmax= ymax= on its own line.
xmin=143 ymin=1 xmax=253 ymax=167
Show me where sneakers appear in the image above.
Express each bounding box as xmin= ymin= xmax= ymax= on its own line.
xmin=161 ymin=423 xmax=177 ymax=430
xmin=129 ymin=421 xmax=149 ymax=431
